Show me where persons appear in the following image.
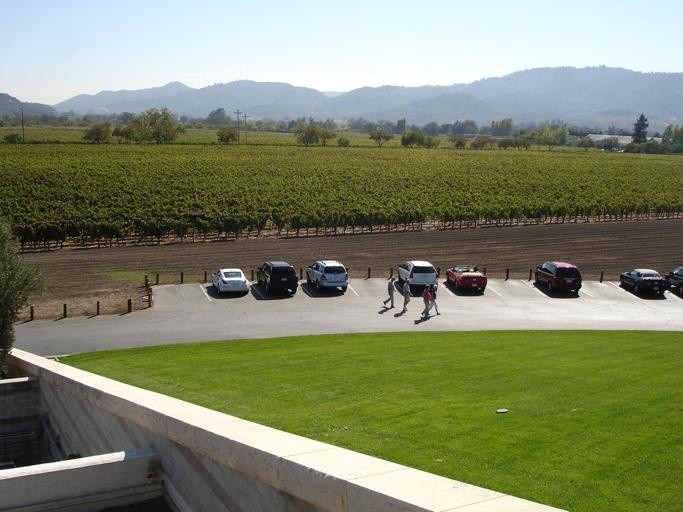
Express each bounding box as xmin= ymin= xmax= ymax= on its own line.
xmin=421 ymin=285 xmax=432 ymax=318
xmin=403 ymin=279 xmax=413 ymax=313
xmin=429 ymin=285 xmax=440 ymax=315
xmin=382 ymin=276 xmax=397 ymax=308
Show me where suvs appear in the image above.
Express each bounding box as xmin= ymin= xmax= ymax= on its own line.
xmin=305 ymin=260 xmax=348 ymax=293
xmin=397 ymin=261 xmax=437 ymax=286
xmin=256 ymin=260 xmax=298 ymax=294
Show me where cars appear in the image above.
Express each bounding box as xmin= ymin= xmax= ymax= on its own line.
xmin=620 ymin=268 xmax=669 ymax=297
xmin=664 ymin=266 xmax=683 ymax=299
xmin=210 ymin=268 xmax=251 ymax=295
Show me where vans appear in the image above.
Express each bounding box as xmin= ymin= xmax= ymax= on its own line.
xmin=534 ymin=261 xmax=581 ymax=292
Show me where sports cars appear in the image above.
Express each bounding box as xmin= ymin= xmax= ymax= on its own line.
xmin=446 ymin=266 xmax=487 ymax=294
